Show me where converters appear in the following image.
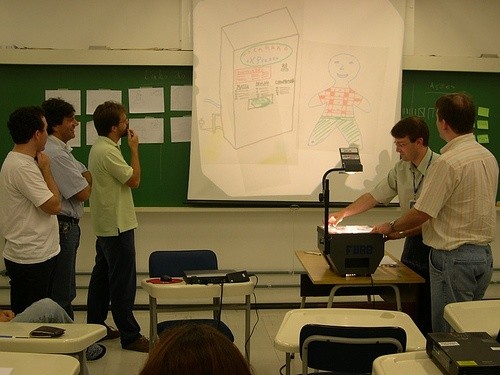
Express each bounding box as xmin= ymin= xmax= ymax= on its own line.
xmin=225 ymin=270 xmax=252 ymax=283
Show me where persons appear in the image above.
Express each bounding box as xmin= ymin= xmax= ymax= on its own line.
xmin=87 ymin=102 xmax=150 ymax=352
xmin=322 ymin=116 xmax=440 ymax=335
xmin=0 ymin=298 xmax=107 ymax=361
xmin=0 ymin=106 xmax=62 ymax=316
xmin=43 ymin=98 xmax=92 ymax=320
xmin=370 ymin=96 xmax=500 ymax=331
xmin=138 ymin=324 xmax=252 ymax=375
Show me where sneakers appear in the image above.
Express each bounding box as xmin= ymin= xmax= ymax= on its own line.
xmin=122 ymin=334 xmax=149 ymax=353
xmin=97 ymin=323 xmax=120 ymax=342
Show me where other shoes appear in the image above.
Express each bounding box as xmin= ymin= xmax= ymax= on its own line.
xmin=86 ymin=344 xmax=107 ymax=362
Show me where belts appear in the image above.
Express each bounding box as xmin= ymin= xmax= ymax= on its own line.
xmin=57 ymin=215 xmax=79 ymax=224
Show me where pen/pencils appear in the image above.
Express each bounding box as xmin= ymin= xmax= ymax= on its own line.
xmin=304 ymin=252 xmax=321 ymax=256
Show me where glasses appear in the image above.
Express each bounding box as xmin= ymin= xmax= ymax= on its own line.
xmin=118 ymin=118 xmax=128 ymax=124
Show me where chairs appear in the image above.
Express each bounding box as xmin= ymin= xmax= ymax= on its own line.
xmin=296 ymin=323 xmax=407 ymax=375
xmin=147 ymin=250 xmax=236 ymax=356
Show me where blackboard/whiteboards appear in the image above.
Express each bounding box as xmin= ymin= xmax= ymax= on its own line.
xmin=0 ymin=64 xmax=500 ymax=208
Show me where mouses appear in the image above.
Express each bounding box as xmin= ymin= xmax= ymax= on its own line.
xmin=160 ymin=274 xmax=172 ymax=282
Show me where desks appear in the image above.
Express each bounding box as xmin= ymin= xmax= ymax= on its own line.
xmin=0 ymin=320 xmax=108 ymax=375
xmin=293 ymin=249 xmax=425 ymax=313
xmin=141 ymin=274 xmax=254 ymax=372
xmin=273 ymin=299 xmax=500 ymax=375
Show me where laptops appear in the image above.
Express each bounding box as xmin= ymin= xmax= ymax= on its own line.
xmin=182 ymin=270 xmax=228 ymax=284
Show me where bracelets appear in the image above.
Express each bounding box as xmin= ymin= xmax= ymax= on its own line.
xmin=400 ymin=232 xmax=405 ymax=238
xmin=390 ymin=220 xmax=397 ymax=232
